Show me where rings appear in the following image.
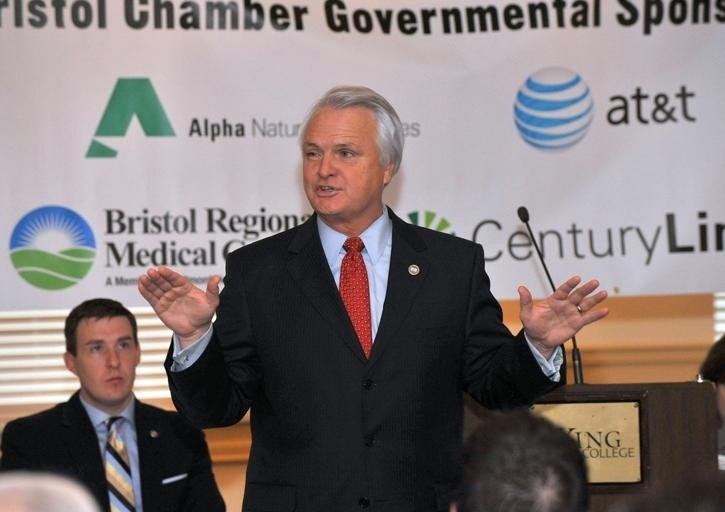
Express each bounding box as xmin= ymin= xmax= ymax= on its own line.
xmin=576 ymin=305 xmax=583 ymax=313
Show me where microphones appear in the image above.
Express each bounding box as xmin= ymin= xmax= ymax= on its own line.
xmin=517 ymin=206 xmax=586 ymax=385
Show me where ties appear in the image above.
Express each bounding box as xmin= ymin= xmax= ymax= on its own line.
xmin=102 ymin=418 xmax=135 ymax=512
xmin=339 ymin=237 xmax=372 ymax=359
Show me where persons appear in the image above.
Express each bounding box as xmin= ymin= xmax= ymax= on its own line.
xmin=450 ymin=410 xmax=590 ymax=512
xmin=135 ymin=84 xmax=611 ymax=512
xmin=2 ymin=299 xmax=225 ymax=512
xmin=701 ymin=332 xmax=725 ymax=426
xmin=2 ymin=471 xmax=101 ymax=511
